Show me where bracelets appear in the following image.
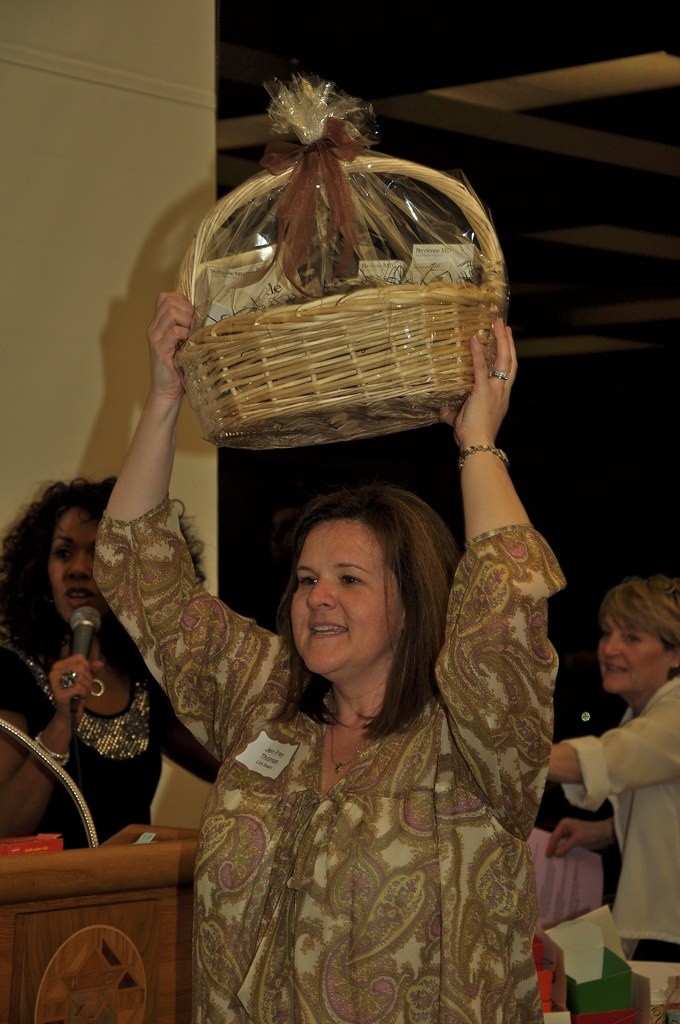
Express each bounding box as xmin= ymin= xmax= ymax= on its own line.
xmin=456 ymin=443 xmax=511 ymax=474
xmin=36 ymin=732 xmax=70 ymax=767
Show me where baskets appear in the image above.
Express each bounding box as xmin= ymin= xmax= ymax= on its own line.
xmin=166 ymin=153 xmax=508 ymax=448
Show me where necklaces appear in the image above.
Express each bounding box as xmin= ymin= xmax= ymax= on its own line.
xmin=83 ymin=648 xmax=106 ymax=698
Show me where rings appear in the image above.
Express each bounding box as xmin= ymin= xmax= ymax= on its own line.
xmin=59 ymin=670 xmax=75 ymax=689
xmin=489 ymin=370 xmax=510 ymax=381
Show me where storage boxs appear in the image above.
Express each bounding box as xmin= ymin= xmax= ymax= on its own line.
xmin=530 ymin=905 xmax=680 ymax=1024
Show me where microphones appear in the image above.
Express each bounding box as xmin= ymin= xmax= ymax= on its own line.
xmin=68 ymin=606 xmax=101 ymax=714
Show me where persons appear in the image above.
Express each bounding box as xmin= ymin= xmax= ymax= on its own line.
xmin=544 ymin=575 xmax=679 ymax=960
xmin=94 ymin=292 xmax=565 ymax=1024
xmin=0 ymin=479 xmax=225 ymax=850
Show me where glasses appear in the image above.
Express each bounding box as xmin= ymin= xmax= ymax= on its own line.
xmin=622 ymin=576 xmax=680 ymax=598
